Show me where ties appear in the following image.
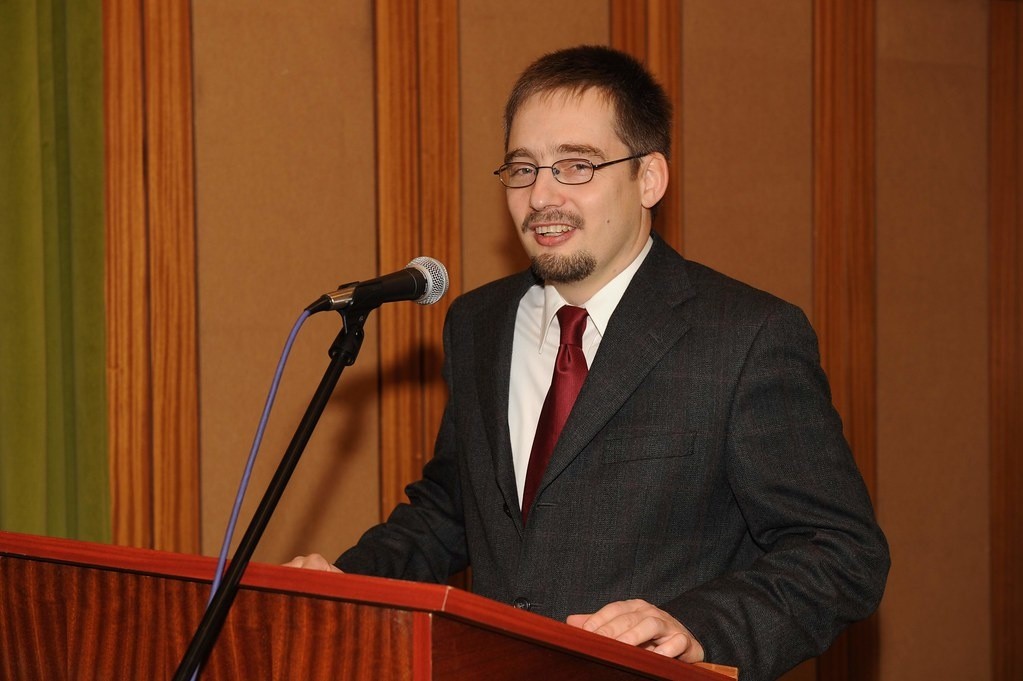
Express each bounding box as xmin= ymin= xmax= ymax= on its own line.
xmin=521 ymin=304 xmax=590 ymax=530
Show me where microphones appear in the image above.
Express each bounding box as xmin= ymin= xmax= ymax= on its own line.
xmin=303 ymin=256 xmax=449 ymax=317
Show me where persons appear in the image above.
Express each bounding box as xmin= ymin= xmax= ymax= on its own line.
xmin=280 ymin=44 xmax=893 ymax=681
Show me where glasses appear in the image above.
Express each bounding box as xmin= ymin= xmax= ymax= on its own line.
xmin=494 ymin=152 xmax=647 ymax=189
